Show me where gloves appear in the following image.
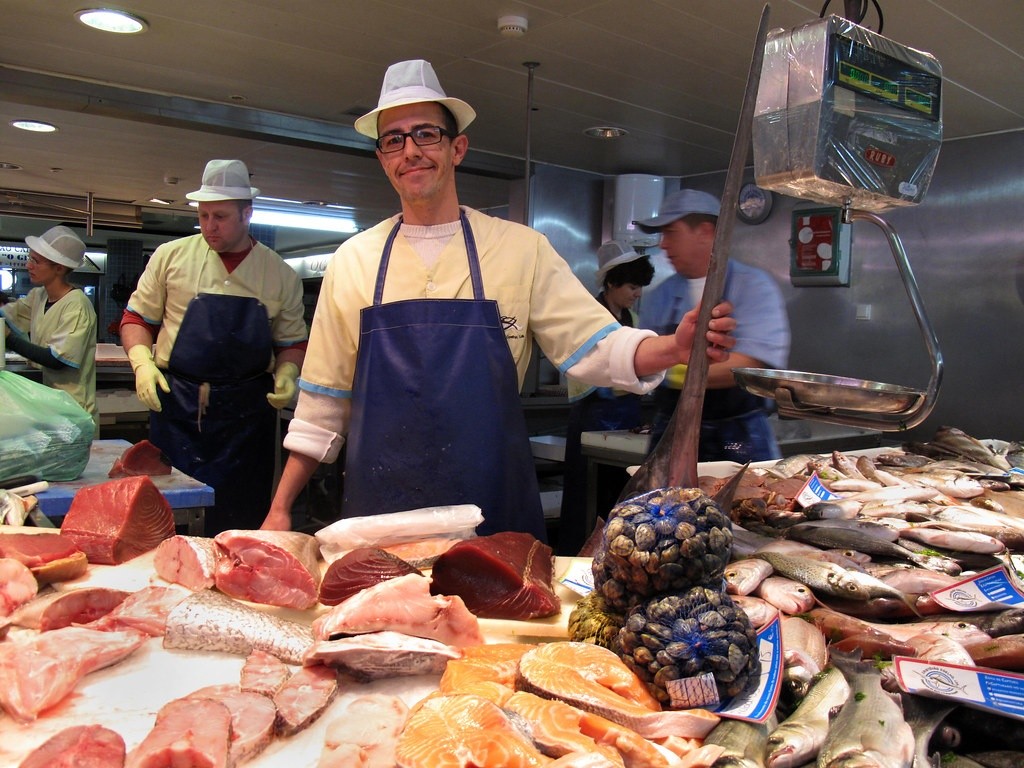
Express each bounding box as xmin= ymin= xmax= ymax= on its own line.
xmin=266 ymin=361 xmax=299 ymax=410
xmin=127 ymin=345 xmax=171 ymax=412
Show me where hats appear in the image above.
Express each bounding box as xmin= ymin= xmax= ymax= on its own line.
xmin=186 ymin=160 xmax=261 ymax=202
xmin=636 ymin=189 xmax=721 ymax=234
xmin=354 ymin=60 xmax=477 ymax=139
xmin=25 ymin=226 xmax=86 ymax=269
xmin=594 ymin=239 xmax=651 ymax=288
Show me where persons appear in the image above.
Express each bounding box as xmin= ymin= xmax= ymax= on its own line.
xmin=259 ymin=60 xmax=737 ymax=550
xmin=560 ymin=237 xmax=655 ymax=547
xmin=633 ymin=189 xmax=790 ymax=463
xmin=0 ymin=225 xmax=100 ymax=440
xmin=118 ymin=160 xmax=309 ymax=539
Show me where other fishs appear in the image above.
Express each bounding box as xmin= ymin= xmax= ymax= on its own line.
xmin=694 ymin=424 xmax=1024 ymax=767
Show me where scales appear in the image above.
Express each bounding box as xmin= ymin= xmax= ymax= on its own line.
xmin=727 ymin=12 xmax=946 ymax=434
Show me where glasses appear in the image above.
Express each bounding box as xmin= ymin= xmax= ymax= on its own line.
xmin=375 ymin=126 xmax=458 ymax=154
xmin=24 ymin=251 xmax=59 ymax=266
